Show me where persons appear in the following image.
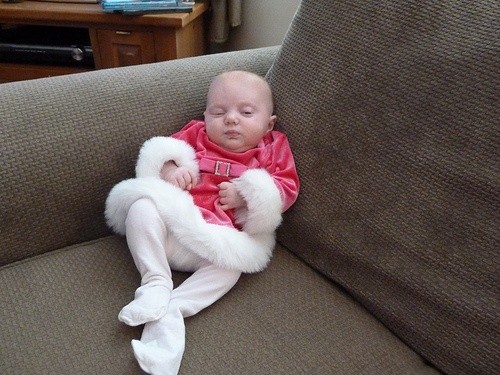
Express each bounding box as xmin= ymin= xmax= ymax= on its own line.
xmin=99 ymin=69 xmax=300 ymax=375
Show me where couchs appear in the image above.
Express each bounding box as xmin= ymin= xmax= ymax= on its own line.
xmin=0 ymin=3 xmax=500 ymax=375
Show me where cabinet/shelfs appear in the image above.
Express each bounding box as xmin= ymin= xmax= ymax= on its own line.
xmin=0 ymin=0 xmax=210 ymax=86
